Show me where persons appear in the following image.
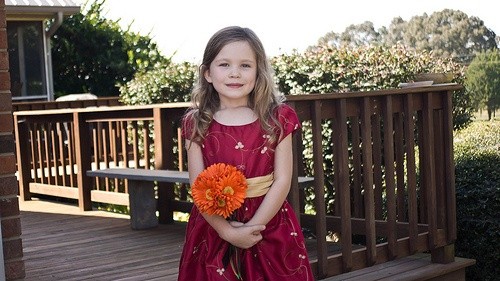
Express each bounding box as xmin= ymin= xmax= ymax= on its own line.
xmin=177 ymin=26 xmax=314 ymax=281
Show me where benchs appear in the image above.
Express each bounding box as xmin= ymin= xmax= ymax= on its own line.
xmin=87 ymin=167 xmax=315 ymax=230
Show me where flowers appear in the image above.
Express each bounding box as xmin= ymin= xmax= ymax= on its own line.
xmin=191 ymin=163 xmax=249 ymax=219
